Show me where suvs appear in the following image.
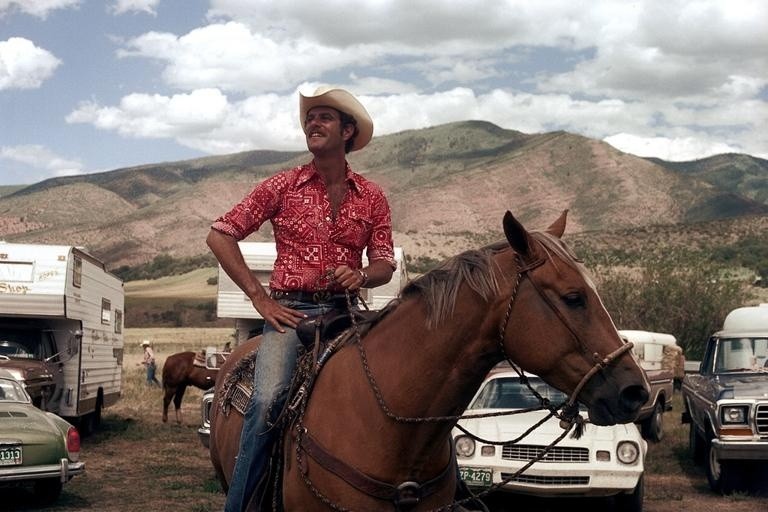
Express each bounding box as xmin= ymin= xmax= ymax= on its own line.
xmin=615 ymin=330 xmax=680 ymax=444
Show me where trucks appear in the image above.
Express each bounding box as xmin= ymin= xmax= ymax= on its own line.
xmin=0 ymin=239 xmax=126 ymax=449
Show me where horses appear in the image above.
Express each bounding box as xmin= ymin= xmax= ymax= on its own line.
xmin=158 ymin=350 xmax=237 ymax=423
xmin=206 ymin=205 xmax=653 ymax=512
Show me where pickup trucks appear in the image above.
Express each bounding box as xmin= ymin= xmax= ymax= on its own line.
xmin=679 ymin=327 xmax=768 ymax=497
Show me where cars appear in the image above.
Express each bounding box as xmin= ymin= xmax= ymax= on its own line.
xmin=0 ymin=364 xmax=88 ymax=508
xmin=449 ymin=354 xmax=649 ymax=512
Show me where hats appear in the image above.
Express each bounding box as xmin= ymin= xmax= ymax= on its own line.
xmin=297 ymin=85 xmax=374 ymax=152
xmin=139 ymin=340 xmax=152 ymax=347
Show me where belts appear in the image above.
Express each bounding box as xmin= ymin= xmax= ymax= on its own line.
xmin=271 ymin=288 xmax=359 ymax=304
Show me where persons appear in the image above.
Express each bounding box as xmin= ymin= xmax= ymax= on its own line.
xmin=139 ymin=341 xmax=162 ymax=389
xmin=205 ymin=85 xmax=397 ymax=512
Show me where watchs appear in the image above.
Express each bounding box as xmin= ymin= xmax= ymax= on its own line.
xmin=357 ymin=269 xmax=368 ymax=287
